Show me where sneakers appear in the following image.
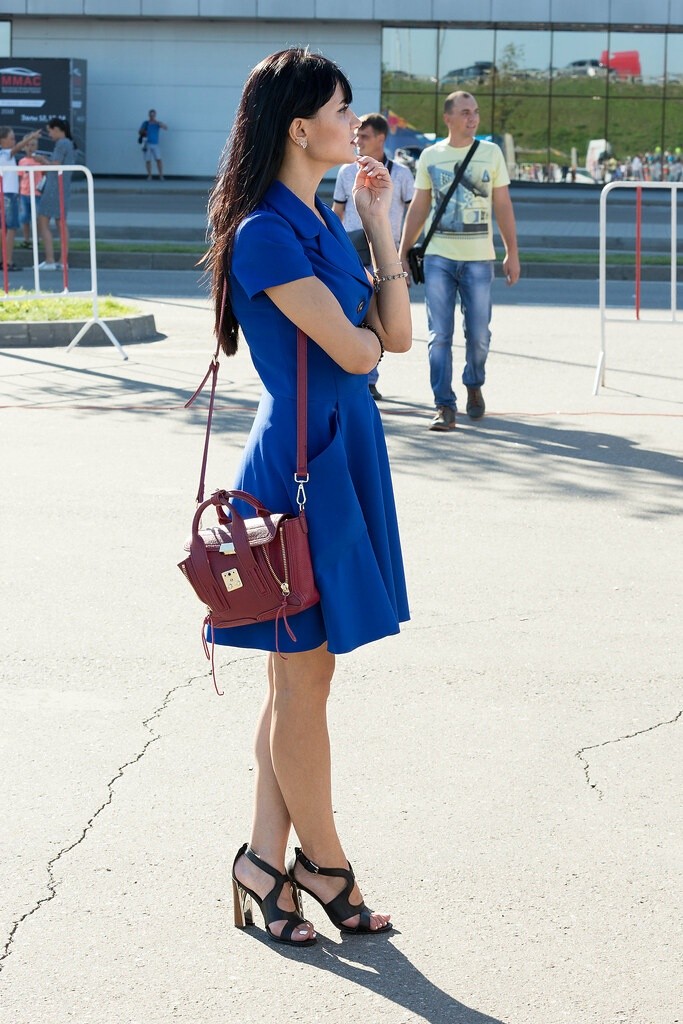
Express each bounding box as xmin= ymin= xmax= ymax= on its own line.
xmin=466 ymin=388 xmax=485 ymax=421
xmin=429 ymin=406 xmax=456 ymax=430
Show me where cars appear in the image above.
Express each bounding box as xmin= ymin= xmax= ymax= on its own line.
xmin=563 ymin=57 xmax=619 ymax=79
xmin=438 ymin=60 xmax=501 ymax=88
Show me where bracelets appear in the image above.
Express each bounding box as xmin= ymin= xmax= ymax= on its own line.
xmin=373 ymin=261 xmax=409 ymax=292
xmin=359 ymin=320 xmax=385 ymax=372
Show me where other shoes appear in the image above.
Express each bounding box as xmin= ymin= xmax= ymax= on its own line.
xmin=57 ymin=262 xmax=68 ymax=270
xmin=7 ymin=263 xmax=23 ymax=271
xmin=369 ymin=385 xmax=382 ymax=401
xmin=147 ymin=175 xmax=153 ymax=181
xmin=21 ymin=240 xmax=39 ymax=249
xmin=33 ymin=260 xmax=57 ymax=270
xmin=160 ymin=175 xmax=164 ymax=181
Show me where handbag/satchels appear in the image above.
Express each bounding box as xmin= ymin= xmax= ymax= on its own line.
xmin=407 ymin=242 xmax=425 ymax=284
xmin=178 ymin=489 xmax=319 ymax=695
xmin=35 ymin=175 xmax=47 ymax=192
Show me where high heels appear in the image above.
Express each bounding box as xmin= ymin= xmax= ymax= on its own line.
xmin=286 ymin=846 xmax=393 ymax=934
xmin=231 ymin=842 xmax=317 ymax=947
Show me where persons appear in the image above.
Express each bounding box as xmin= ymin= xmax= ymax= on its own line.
xmin=138 ymin=109 xmax=168 ymax=182
xmin=0 ymin=125 xmax=42 ymax=272
xmin=14 ymin=131 xmax=48 ymax=250
xmin=594 ymin=145 xmax=683 ymax=185
xmin=30 ymin=117 xmax=78 ymax=271
xmin=398 ymin=91 xmax=521 ymax=432
xmin=510 ymin=162 xmax=576 ymax=183
xmin=331 ymin=113 xmax=416 ymax=401
xmin=203 ymin=48 xmax=413 ymax=947
